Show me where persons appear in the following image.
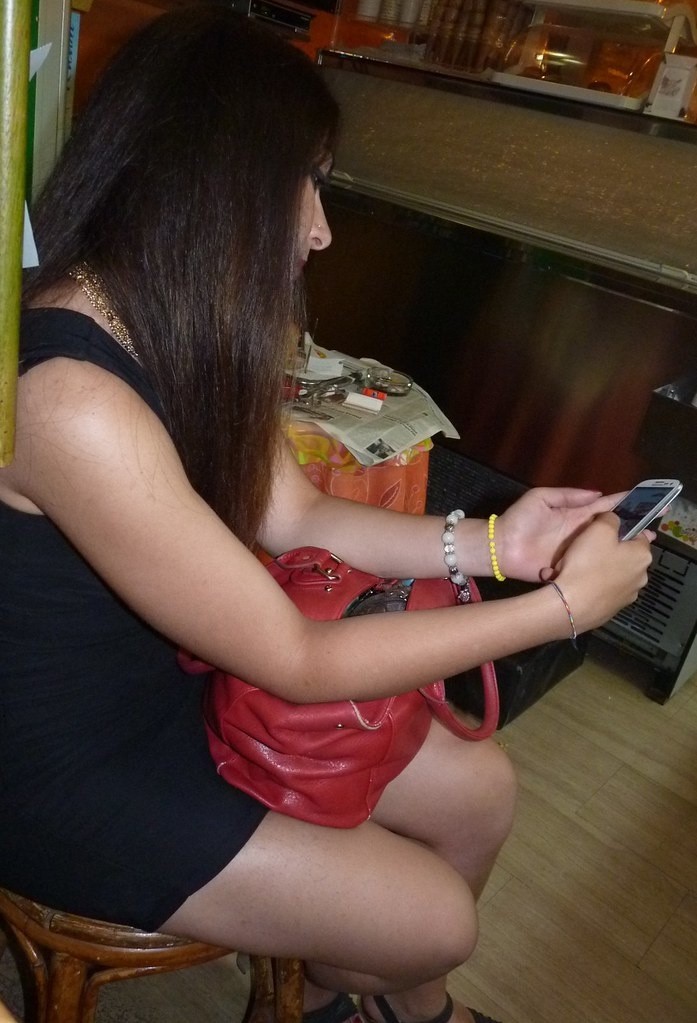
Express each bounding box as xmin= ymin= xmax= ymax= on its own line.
xmin=0 ymin=16 xmax=655 ymax=1023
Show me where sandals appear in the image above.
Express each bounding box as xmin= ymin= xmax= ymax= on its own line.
xmin=362 ymin=991 xmax=501 ymax=1023
xmin=238 ymin=953 xmax=362 ymax=1022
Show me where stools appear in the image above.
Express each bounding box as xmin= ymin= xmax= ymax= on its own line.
xmin=0 ymin=888 xmax=305 ymax=1022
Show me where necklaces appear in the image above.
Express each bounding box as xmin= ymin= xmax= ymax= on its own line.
xmin=69 ymin=260 xmax=140 ymax=357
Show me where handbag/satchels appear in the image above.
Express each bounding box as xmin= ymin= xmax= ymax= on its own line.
xmin=180 ymin=546 xmax=500 ymax=831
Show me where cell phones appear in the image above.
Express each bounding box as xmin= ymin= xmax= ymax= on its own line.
xmin=555 ymin=478 xmax=683 ymax=573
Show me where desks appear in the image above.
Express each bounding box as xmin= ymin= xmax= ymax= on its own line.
xmin=281 ymin=350 xmax=434 ymax=516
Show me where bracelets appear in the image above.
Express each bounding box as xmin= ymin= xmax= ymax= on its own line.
xmin=443 ymin=509 xmax=471 ymax=602
xmin=545 ymin=580 xmax=576 ymax=640
xmin=488 ymin=513 xmax=506 ymax=581
